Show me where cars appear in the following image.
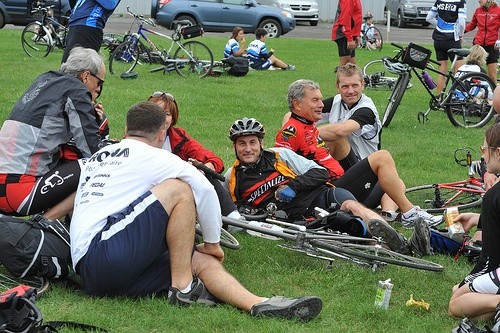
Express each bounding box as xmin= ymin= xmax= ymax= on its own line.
xmin=1 ymin=0 xmax=77 ymax=28
xmin=151 ymin=0 xmax=298 ymax=39
xmin=253 ymin=0 xmax=319 ymax=26
xmin=384 ymin=0 xmax=437 ymax=30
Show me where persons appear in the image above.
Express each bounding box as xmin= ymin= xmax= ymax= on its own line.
xmin=360 ymin=12 xmax=375 ymax=47
xmin=224 ymin=27 xmax=246 ymax=58
xmin=464 ymin=0 xmax=500 ymax=86
xmin=275 ymin=79 xmax=443 ymax=229
xmin=62 ymin=0 xmax=121 ymax=64
xmin=426 ymin=0 xmax=467 ymax=95
xmin=283 ymin=64 xmax=382 ymax=171
xmin=148 ymin=92 xmax=246 ymax=232
xmin=0 ymin=46 xmax=111 ymax=223
xmin=70 ymin=102 xmax=322 ymax=323
xmin=222 ymin=117 xmax=434 ymax=260
xmin=247 ymin=29 xmax=295 ymax=71
xmin=450 ymin=122 xmax=500 ymax=332
xmin=456 ymin=44 xmax=493 ymax=100
xmin=331 ymin=0 xmax=362 ymax=70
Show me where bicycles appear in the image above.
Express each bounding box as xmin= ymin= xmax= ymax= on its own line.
xmin=397 ymin=147 xmax=491 ymax=213
xmin=362 ymin=58 xmax=414 ymax=91
xmin=355 ymin=26 xmax=382 ymax=51
xmin=21 ymin=3 xmax=120 ymax=58
xmin=108 ymin=5 xmax=233 ymax=80
xmin=196 ymin=210 xmax=446 ymax=275
xmin=0 ymin=262 xmax=50 ymax=297
xmin=377 ymin=39 xmax=496 ymax=133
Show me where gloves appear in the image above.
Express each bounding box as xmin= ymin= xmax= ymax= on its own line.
xmin=494 ymin=40 xmax=500 ymax=50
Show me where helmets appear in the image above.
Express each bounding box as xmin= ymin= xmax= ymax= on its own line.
xmin=0 ymin=291 xmax=42 ymax=333
xmin=229 ymin=117 xmax=265 ymax=141
xmin=363 ymin=12 xmax=373 ymax=19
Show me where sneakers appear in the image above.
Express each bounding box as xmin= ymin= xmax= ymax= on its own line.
xmin=407 ymin=217 xmax=434 ymax=256
xmin=282 ymin=65 xmax=295 ymax=71
xmin=402 ymin=206 xmax=446 ymax=229
xmin=167 ymin=277 xmax=225 ymax=307
xmin=250 ymin=295 xmax=323 ymax=323
xmin=379 ymin=210 xmax=402 ymax=222
xmin=368 ymin=219 xmax=410 ymax=256
xmin=452 ymin=318 xmax=487 ymax=333
xmin=491 ymin=302 xmax=500 ymax=333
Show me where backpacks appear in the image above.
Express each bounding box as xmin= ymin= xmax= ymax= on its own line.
xmin=0 ymin=213 xmax=71 ymax=282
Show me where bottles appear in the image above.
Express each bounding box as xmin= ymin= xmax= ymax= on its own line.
xmin=420 ymin=71 xmax=437 ymax=89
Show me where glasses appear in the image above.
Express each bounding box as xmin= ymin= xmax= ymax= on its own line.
xmin=77 ymin=70 xmax=105 ymax=87
xmin=480 ymin=145 xmax=496 ymax=154
xmin=147 ymin=91 xmax=174 ymax=102
